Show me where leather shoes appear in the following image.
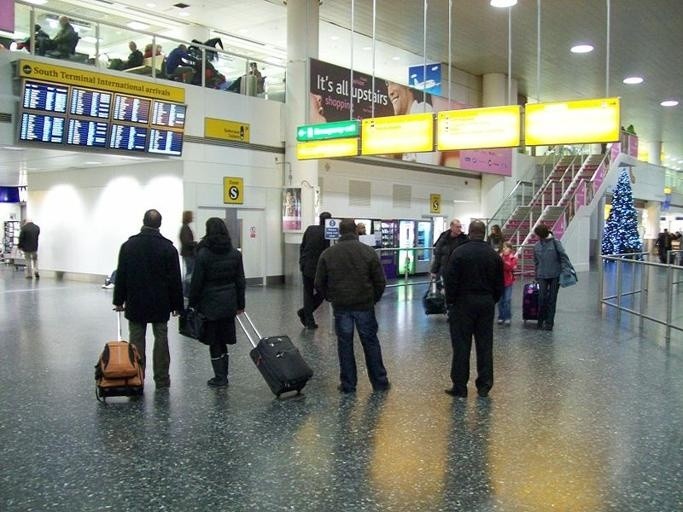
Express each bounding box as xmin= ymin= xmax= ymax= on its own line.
xmin=297 ymin=309 xmax=320 ymax=329
xmin=477 ymin=390 xmax=490 ymax=398
xmin=444 ymin=387 xmax=468 ymax=398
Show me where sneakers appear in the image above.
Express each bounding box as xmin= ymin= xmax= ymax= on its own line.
xmin=497 ymin=318 xmax=504 ymax=325
xmin=34 ymin=272 xmax=39 ymax=278
xmin=504 ymin=319 xmax=511 ymax=326
xmin=25 ymin=275 xmax=32 ymax=279
xmin=372 ymin=383 xmax=391 ymax=391
xmin=335 ymin=383 xmax=357 ymax=394
xmin=153 ymin=376 xmax=171 ymax=390
xmin=101 ymin=278 xmax=115 ymax=289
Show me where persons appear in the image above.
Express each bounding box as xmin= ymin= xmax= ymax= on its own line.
xmin=356 ymin=223 xmax=366 ymax=235
xmin=179 ymin=210 xmax=198 ymax=310
xmin=18 ymin=15 xmax=264 ymax=95
xmin=487 ymin=224 xmax=504 ymax=255
xmin=17 ymin=217 xmax=40 ymax=278
xmin=309 ymin=92 xmax=326 ymax=124
xmin=313 ymin=218 xmax=389 ymax=393
xmin=297 ymin=212 xmax=332 ymax=330
xmin=655 ymin=229 xmax=683 ymax=267
xmin=444 ymin=220 xmax=504 ymax=397
xmin=532 ymin=224 xmax=564 ymax=332
xmin=497 ymin=241 xmax=518 ymax=327
xmin=430 ymin=220 xmax=468 ymax=323
xmin=112 ymin=210 xmax=183 ymax=389
xmin=384 ymin=80 xmax=442 ymax=166
xmin=189 ymin=218 xmax=245 ymax=388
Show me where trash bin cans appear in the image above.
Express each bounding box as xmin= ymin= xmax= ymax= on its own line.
xmin=240 ymin=75 xmax=258 ymax=97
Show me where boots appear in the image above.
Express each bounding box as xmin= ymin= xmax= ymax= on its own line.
xmin=206 ymin=352 xmax=230 ymax=388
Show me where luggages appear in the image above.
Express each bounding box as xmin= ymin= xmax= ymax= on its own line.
xmin=522 ymin=280 xmax=542 ymax=325
xmin=234 ymin=310 xmax=314 ymax=401
xmin=93 ymin=307 xmax=145 ymax=404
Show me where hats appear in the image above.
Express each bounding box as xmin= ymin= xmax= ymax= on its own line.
xmin=534 ymin=224 xmax=550 ymax=239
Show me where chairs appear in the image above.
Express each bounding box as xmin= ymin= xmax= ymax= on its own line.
xmin=0 ymin=26 xmax=286 ymax=107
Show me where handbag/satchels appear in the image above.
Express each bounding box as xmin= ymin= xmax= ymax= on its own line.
xmin=553 ymin=238 xmax=579 ymax=290
xmin=176 ymin=301 xmax=216 ymax=347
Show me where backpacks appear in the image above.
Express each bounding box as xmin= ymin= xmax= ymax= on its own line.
xmin=422 ymin=273 xmax=448 ymax=316
